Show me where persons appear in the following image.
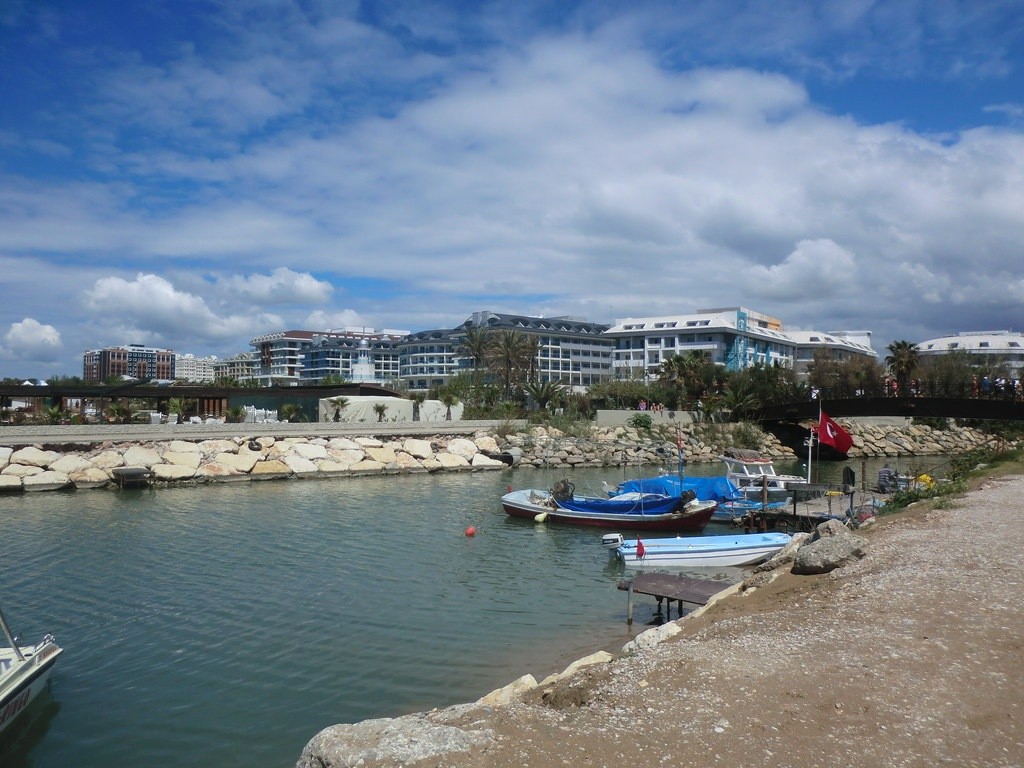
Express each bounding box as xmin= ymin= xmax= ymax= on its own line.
xmin=639 ymin=399 xmax=664 ymax=412
xmin=885 ymin=379 xmax=919 ymax=397
xmin=878 ymin=464 xmax=896 ymax=494
xmin=980 ymin=376 xmax=1022 ymax=400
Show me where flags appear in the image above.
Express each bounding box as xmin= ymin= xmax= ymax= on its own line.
xmin=725 ymin=337 xmax=770 ymax=372
xmin=637 ymin=534 xmax=646 ymax=558
xmin=820 ymin=410 xmax=854 ymax=454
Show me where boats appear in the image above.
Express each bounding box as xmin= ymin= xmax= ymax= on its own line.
xmin=0 ymin=609 xmax=65 ymax=731
xmin=501 ymin=476 xmax=719 ymax=532
xmin=600 ymin=532 xmax=795 ymax=567
xmin=658 ymin=447 xmax=808 ymax=492
xmin=600 ymin=473 xmax=793 ymax=521
xmin=878 ymin=462 xmax=951 ymax=493
